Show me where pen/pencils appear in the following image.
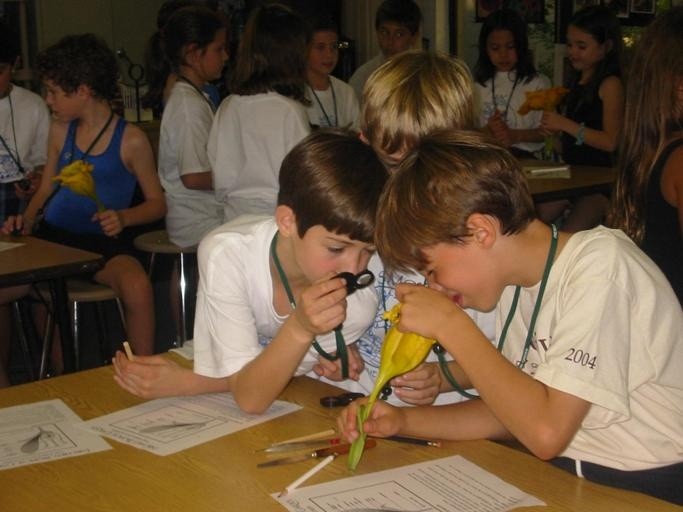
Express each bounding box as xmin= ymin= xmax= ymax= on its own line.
xmin=254 ymin=429 xmax=441 ymax=498
xmin=123 ymin=340 xmax=134 ymax=361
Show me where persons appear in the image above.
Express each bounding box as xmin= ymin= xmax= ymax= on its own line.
xmin=335 ymin=128 xmax=682 ymax=504
xmin=305 ymin=48 xmax=498 ymax=410
xmin=112 ymin=131 xmax=390 ymax=415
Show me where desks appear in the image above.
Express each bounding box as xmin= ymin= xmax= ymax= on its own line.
xmin=0 ymin=346 xmax=682 ymax=511
xmin=505 ymin=151 xmax=623 ymax=202
xmin=0 ymin=227 xmax=107 ymax=384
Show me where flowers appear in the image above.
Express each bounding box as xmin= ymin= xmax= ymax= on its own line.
xmin=45 ymin=159 xmax=120 ymax=242
xmin=511 ymin=82 xmax=573 ymax=162
xmin=342 ymin=280 xmax=447 ymax=472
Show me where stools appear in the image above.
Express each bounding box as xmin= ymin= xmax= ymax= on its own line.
xmin=126 ymin=224 xmax=202 ymax=352
xmin=25 ymin=274 xmax=134 ymax=386
xmin=0 ymin=281 xmax=37 ymax=389
